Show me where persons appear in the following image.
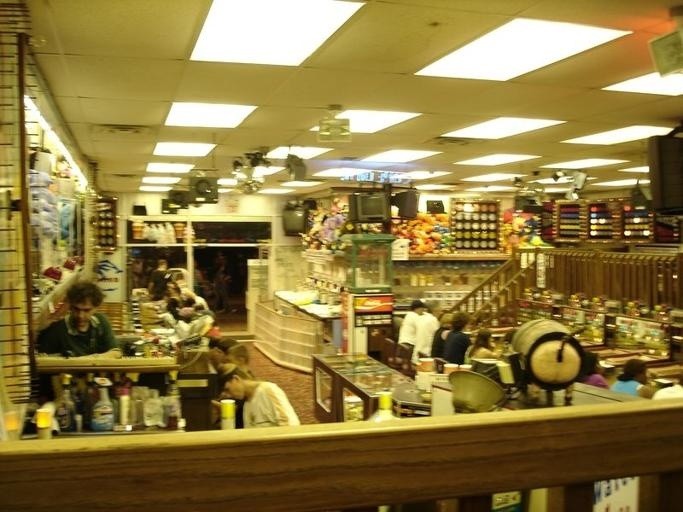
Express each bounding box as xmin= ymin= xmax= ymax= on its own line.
xmin=608 ymin=357 xmax=652 ymax=399
xmin=129 ymin=249 xmax=300 ymax=428
xmin=397 ymin=298 xmax=504 ymax=366
xmin=651 ymin=374 xmax=682 ymax=399
xmin=37 ymin=280 xmax=120 ymax=399
xmin=581 ymin=351 xmax=607 ymax=389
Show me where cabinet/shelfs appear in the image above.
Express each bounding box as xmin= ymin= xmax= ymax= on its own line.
xmin=308 ymin=351 xmax=413 ymax=424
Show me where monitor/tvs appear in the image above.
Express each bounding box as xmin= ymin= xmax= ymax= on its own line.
xmin=281 ymin=208 xmax=307 ymax=236
xmin=647 ymin=135 xmax=683 ymax=216
xmin=397 ymin=191 xmax=417 ymax=218
xmin=349 ymin=193 xmax=391 ymax=224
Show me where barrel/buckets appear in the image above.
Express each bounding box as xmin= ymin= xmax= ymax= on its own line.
xmin=510 ymin=317 xmax=585 ymax=392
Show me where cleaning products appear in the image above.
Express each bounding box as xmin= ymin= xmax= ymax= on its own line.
xmin=53 ymin=373 xmax=76 ymax=432
xmin=119 ymin=386 xmax=130 ymax=425
xmin=89 ymin=377 xmax=115 ymax=432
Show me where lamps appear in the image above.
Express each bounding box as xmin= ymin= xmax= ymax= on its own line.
xmin=22 ymin=32 xmax=47 ymax=49
xmin=162 ymin=102 xmax=352 ymax=212
